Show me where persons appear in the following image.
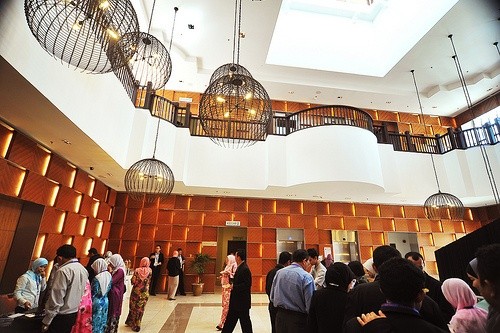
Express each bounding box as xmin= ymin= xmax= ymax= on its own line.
xmin=321 ymin=254 xmax=334 ymax=270
xmin=216 ymin=255 xmax=237 ymax=330
xmin=307 ymin=248 xmax=327 ymax=291
xmin=167 ymin=251 xmax=181 ymax=301
xmin=148 ymin=245 xmax=164 ymax=296
xmin=304 ymin=245 xmax=500 ymax=333
xmin=14 ymin=258 xmax=48 ymax=314
xmin=91 ymin=258 xmax=113 ymax=333
xmin=41 ymin=245 xmax=93 ymax=333
xmin=103 ymin=254 xmax=126 ymax=333
xmin=266 ymin=251 xmax=293 ymax=333
xmin=176 ymin=248 xmax=186 ymax=296
xmin=221 ymin=249 xmax=253 ymax=333
xmin=87 ymin=248 xmax=104 ymax=282
xmin=270 ymin=249 xmax=315 ymax=333
xmin=125 ymin=256 xmax=152 ymax=332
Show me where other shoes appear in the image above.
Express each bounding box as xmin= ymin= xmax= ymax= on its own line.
xmin=180 ymin=293 xmax=186 ymax=296
xmin=136 ymin=326 xmax=140 ymax=332
xmin=216 ymin=326 xmax=223 ymax=329
xmin=150 ymin=293 xmax=156 ymax=296
xmin=169 ymin=298 xmax=175 ymax=300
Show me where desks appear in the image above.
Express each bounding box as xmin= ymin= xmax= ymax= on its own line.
xmin=0 ymin=308 xmax=41 ymax=333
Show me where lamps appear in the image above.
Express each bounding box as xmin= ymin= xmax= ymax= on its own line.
xmin=411 ymin=69 xmax=464 ymax=221
xmin=199 ymin=0 xmax=273 ymax=148
xmin=23 ymin=0 xmax=175 ymax=203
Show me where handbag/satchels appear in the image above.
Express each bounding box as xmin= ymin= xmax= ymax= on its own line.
xmin=229 ymin=265 xmax=234 ymax=284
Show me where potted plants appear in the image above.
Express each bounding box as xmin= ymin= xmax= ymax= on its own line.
xmin=187 ymin=253 xmax=215 ymax=296
